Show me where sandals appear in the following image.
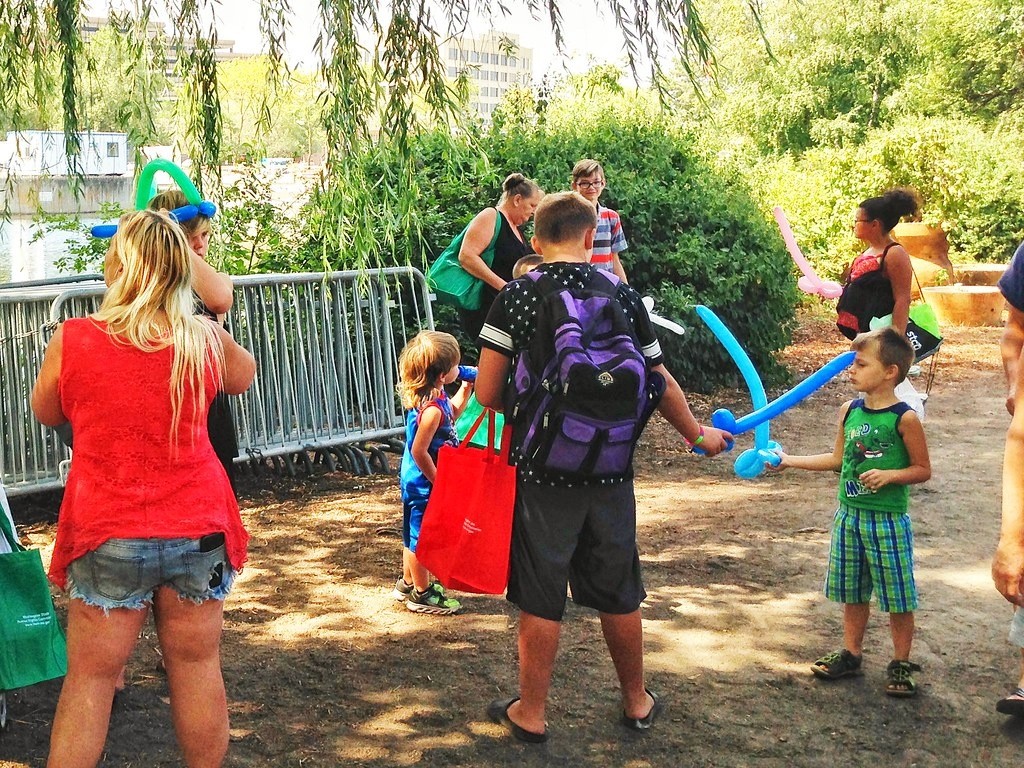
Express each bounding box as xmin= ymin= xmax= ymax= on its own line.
xmin=811 ymin=649 xmax=862 ymax=677
xmin=885 ymin=660 xmax=920 ymax=694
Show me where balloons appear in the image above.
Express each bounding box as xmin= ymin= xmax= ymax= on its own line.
xmin=774 ymin=206 xmax=842 ymax=299
xmin=640 ymin=296 xmax=685 ymax=336
xmin=689 ymin=350 xmax=855 ymax=457
xmin=695 ymin=306 xmax=783 ymax=479
xmin=92 ymin=156 xmax=217 ymax=238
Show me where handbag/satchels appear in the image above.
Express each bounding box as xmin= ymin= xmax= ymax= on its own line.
xmin=425 ymin=207 xmax=501 ymax=310
xmin=416 ymin=407 xmax=517 ymax=594
xmin=870 ymin=305 xmax=943 ymax=363
xmin=0 ymin=501 xmax=68 ymax=689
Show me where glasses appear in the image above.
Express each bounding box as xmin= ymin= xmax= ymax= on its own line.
xmin=575 ymin=180 xmax=602 ymax=188
xmin=851 ymin=219 xmax=873 ymax=226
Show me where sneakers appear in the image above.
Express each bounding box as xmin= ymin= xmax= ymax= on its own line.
xmin=391 ymin=579 xmax=444 ymax=600
xmin=405 ymin=586 xmax=460 ymax=614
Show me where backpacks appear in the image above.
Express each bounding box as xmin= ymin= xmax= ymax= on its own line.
xmin=503 ymin=268 xmax=667 ymax=487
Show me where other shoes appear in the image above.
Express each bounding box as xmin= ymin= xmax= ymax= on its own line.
xmin=996 ymin=687 xmax=1024 ymax=714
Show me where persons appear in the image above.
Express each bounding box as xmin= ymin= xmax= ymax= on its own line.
xmin=571 ymin=159 xmax=629 ymax=289
xmin=391 ymin=330 xmax=478 ymax=615
xmin=142 ymin=192 xmax=240 ymax=499
xmin=819 ymin=188 xmax=923 ymax=338
xmin=473 ymin=191 xmax=734 ymax=743
xmin=761 ymin=328 xmax=931 ymax=695
xmin=995 ymin=240 xmax=1024 ymax=415
xmin=456 ymin=173 xmax=545 ymax=357
xmin=992 ymin=342 xmax=1024 ymax=714
xmin=31 ymin=210 xmax=256 ymax=768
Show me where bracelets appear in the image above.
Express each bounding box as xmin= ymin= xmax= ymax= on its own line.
xmin=687 ymin=424 xmax=703 ymax=446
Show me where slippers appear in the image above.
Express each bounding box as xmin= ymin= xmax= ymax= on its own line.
xmin=622 ymin=688 xmax=660 ymax=728
xmin=487 ymin=698 xmax=551 ymax=741
